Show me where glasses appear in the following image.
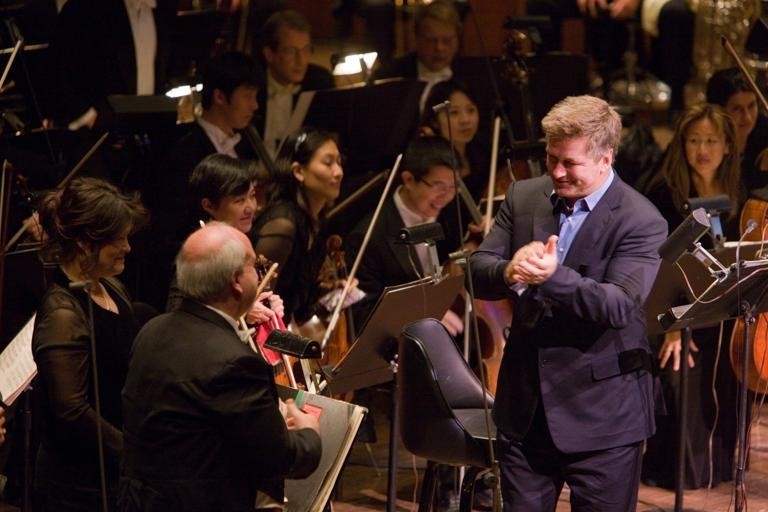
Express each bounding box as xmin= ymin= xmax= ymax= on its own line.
xmin=419 ymin=176 xmax=463 ymax=194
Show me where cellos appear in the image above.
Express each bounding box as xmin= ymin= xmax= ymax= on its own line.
xmin=446 ymin=238 xmax=518 ymax=397
xmin=728 ymin=184 xmax=767 ymax=397
xmin=490 ymin=31 xmax=550 ymax=196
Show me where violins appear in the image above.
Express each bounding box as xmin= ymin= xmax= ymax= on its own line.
xmin=254 ymin=254 xmax=306 ymax=391
xmin=321 ymin=235 xmax=357 ymax=405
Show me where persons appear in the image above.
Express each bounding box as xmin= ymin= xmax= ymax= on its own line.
xmin=33 ymin=179 xmax=147 ymax=511
xmin=119 ymin=220 xmax=323 ymax=511
xmin=0 ymin=0 xmax=766 ymax=512
xmin=464 ymin=95 xmax=670 ymax=510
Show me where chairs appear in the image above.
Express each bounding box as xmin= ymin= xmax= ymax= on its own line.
xmin=397 ymin=318 xmax=500 ymax=512
